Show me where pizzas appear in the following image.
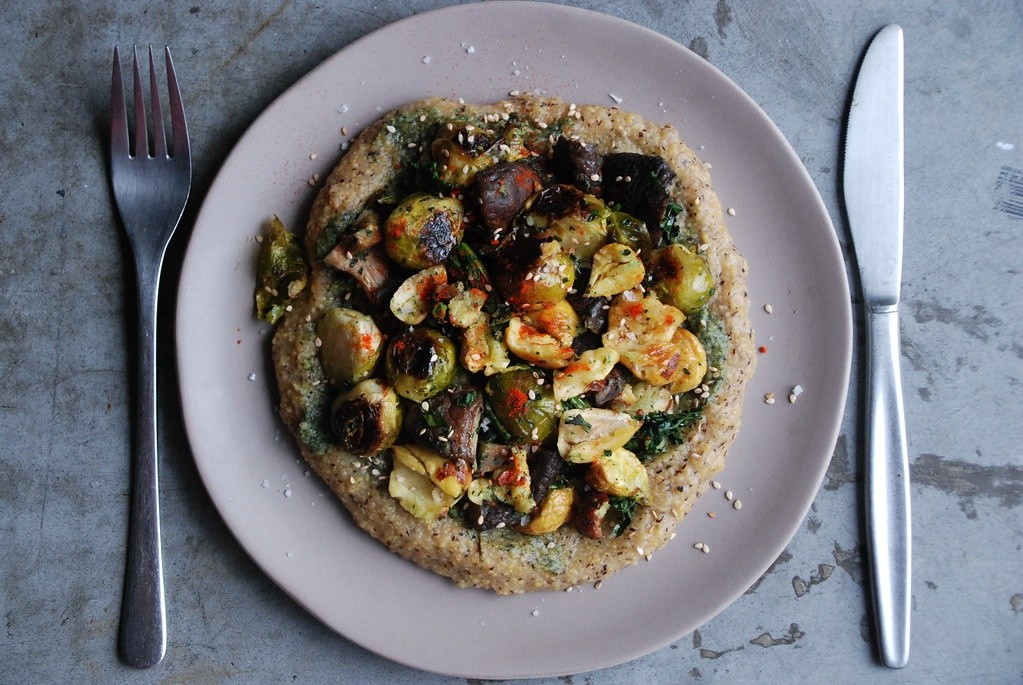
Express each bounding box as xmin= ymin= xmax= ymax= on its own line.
xmin=274 ymin=95 xmax=755 ymax=591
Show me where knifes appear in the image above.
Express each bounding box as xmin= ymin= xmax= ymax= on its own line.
xmin=843 ymin=24 xmax=912 ymax=670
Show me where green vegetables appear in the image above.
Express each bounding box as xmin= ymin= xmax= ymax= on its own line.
xmin=255 ymin=217 xmax=307 ymax=325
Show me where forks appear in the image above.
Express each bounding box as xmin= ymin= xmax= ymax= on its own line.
xmin=109 ymin=45 xmax=191 ymax=671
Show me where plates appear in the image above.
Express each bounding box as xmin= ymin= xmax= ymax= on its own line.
xmin=176 ymin=0 xmax=852 ymax=681
